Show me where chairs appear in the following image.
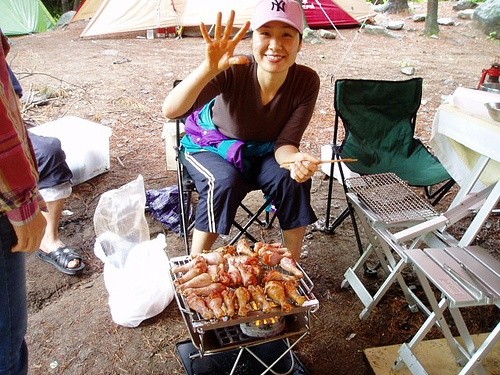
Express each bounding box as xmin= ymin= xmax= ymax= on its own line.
xmin=325 ymin=76 xmax=456 ymax=279
xmin=173 ymin=81 xmax=280 ymax=255
xmin=391 ymin=181 xmax=500 ymax=375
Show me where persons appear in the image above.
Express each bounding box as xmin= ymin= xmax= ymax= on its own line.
xmin=0 ymin=27 xmax=49 ymax=375
xmin=0 ymin=60 xmax=85 ymax=275
xmin=163 ymin=0 xmax=322 ymax=264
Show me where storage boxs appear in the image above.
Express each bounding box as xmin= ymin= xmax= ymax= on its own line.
xmin=321 ymin=143 xmax=359 ymax=188
xmin=26 ymin=116 xmax=113 ymax=188
xmin=161 ymin=122 xmax=183 ymax=171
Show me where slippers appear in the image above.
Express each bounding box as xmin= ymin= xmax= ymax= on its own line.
xmin=36 ymin=244 xmax=85 ymax=274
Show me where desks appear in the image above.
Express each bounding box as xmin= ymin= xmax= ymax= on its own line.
xmin=429 ymin=94 xmax=500 ymax=227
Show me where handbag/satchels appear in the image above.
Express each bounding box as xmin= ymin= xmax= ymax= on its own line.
xmin=92 ymin=232 xmax=174 ymax=328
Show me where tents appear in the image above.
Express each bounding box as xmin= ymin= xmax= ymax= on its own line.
xmin=0 ymin=0 xmax=57 ymax=36
xmin=79 ymin=0 xmax=378 ymax=40
xmin=72 ymin=0 xmax=105 ymax=22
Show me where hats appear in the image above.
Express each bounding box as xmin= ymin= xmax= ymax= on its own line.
xmin=252 ymin=0 xmax=303 ymax=35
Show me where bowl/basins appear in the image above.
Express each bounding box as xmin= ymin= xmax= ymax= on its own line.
xmin=484 ymin=102 xmax=500 ymax=122
xmin=480 ymin=82 xmax=500 ymax=94
